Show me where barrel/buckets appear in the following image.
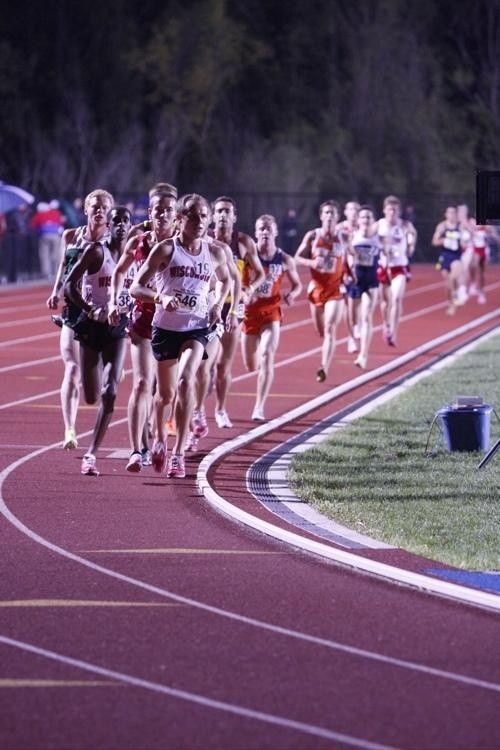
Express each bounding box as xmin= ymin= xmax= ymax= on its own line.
xmin=439 ymin=401 xmax=494 ymax=453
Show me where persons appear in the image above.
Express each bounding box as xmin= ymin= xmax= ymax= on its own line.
xmin=295 ymin=196 xmax=418 ymax=383
xmin=433 ymin=204 xmax=498 ymax=316
xmin=47 ymin=183 xmax=303 ymax=479
xmin=1 ymin=196 xmax=150 ymax=283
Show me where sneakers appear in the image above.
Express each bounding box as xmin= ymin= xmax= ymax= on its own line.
xmin=63 ymin=429 xmax=82 ymax=448
xmin=384 ymin=328 xmax=398 ymax=346
xmin=447 ymin=285 xmax=486 ymax=316
xmin=126 ymin=409 xmax=209 ymax=479
xmin=251 ymin=404 xmax=265 ymax=420
xmin=317 ymin=365 xmax=329 ymax=382
xmin=81 ymin=454 xmax=100 ymax=475
xmin=214 ymin=410 xmax=234 ymax=429
xmin=346 ymin=323 xmax=370 ymax=371
